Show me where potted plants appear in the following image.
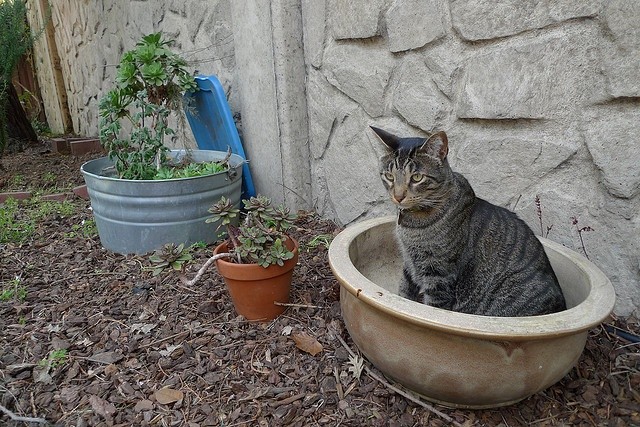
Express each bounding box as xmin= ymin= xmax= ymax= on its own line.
xmin=138 ymin=193 xmax=301 ymax=323
xmin=80 ymin=31 xmax=246 ymax=253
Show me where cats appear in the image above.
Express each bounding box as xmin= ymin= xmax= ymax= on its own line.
xmin=369 ymin=126 xmax=568 ymax=317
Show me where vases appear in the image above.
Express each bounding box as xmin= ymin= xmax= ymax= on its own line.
xmin=326 ymin=214 xmax=616 ymax=411
